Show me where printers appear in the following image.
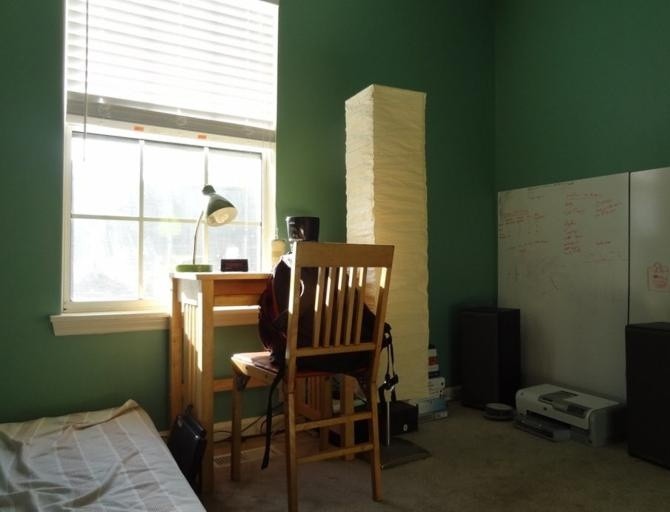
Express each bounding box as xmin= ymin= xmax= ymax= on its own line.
xmin=515 ymin=383 xmax=624 ymax=446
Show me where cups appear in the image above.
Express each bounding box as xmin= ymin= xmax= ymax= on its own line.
xmin=286 ymin=217 xmax=320 ymax=257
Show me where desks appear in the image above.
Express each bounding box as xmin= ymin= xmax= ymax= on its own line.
xmin=168 ymin=271 xmax=331 ymax=494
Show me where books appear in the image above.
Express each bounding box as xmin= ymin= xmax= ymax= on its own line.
xmin=400 ymin=343 xmax=450 ymax=424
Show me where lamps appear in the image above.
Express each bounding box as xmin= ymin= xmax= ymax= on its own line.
xmin=177 ymin=185 xmax=237 ymax=271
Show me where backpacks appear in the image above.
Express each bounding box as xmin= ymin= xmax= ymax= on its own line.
xmin=258 ymin=250 xmax=399 ymax=468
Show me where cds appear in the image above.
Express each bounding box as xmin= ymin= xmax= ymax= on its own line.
xmin=483 ymin=404 xmax=514 ymax=421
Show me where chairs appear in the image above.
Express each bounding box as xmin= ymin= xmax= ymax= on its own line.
xmin=229 ymin=243 xmax=397 ymax=510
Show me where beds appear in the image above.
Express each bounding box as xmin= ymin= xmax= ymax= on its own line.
xmin=1 ymin=401 xmax=207 ymax=510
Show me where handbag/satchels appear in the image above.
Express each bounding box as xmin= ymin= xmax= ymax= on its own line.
xmin=166 ymin=402 xmax=207 ymax=504
